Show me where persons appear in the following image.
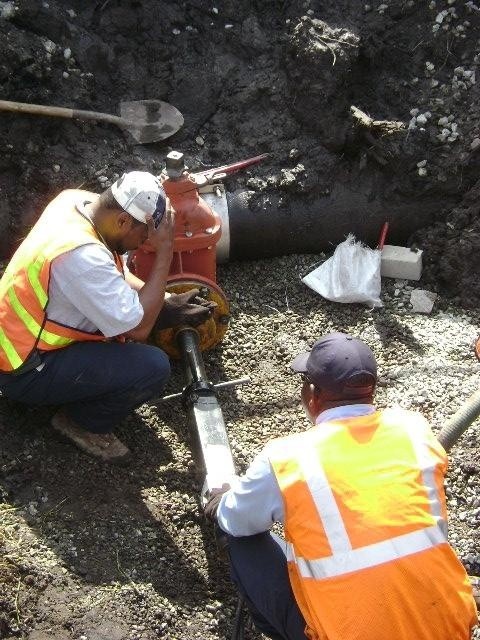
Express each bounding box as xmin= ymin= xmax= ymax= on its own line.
xmin=1 ymin=170 xmax=177 ymax=465
xmin=206 ymin=331 xmax=479 ymax=640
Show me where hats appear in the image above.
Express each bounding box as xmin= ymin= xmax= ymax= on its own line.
xmin=111 ymin=171 xmax=168 ymax=229
xmin=289 ymin=331 xmax=378 ymax=396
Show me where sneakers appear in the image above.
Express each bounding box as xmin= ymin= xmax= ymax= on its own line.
xmin=49 ymin=406 xmax=132 ymax=463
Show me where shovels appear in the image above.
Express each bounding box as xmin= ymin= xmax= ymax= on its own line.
xmin=0 ymin=99 xmax=185 ymax=147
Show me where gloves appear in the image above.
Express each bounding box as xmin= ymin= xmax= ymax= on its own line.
xmin=150 ymin=288 xmax=220 ymax=333
xmin=202 ymin=483 xmax=233 ymax=526
